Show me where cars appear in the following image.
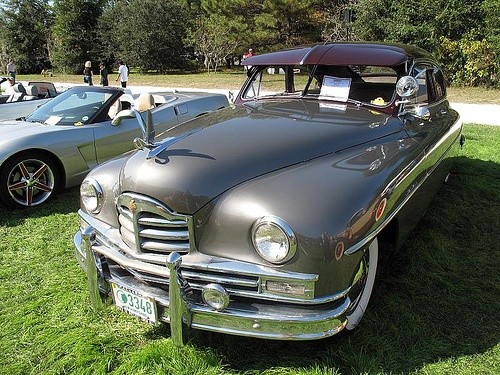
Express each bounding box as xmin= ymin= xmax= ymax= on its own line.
xmin=72 ymin=42 xmax=466 ymax=349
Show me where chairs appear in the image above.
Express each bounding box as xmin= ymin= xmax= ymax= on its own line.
xmin=109 ymin=97 xmax=122 ymax=119
xmin=7 ymin=82 xmax=39 ymax=101
xmin=135 ymin=92 xmax=154 ymax=112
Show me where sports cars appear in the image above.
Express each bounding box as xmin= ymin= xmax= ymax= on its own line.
xmin=0 ymin=85 xmax=230 ymax=212
xmin=0 ymin=81 xmax=66 ymax=123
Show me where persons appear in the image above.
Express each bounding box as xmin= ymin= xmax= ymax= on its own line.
xmin=8 ymin=77 xmax=15 ymax=85
xmin=116 ymin=61 xmax=128 ymax=88
xmin=247 ymin=48 xmax=257 ymax=70
xmin=84 ymin=60 xmax=94 ymax=86
xmin=99 ymin=63 xmax=108 ymax=86
xmin=6 ymin=60 xmax=15 ymax=81
